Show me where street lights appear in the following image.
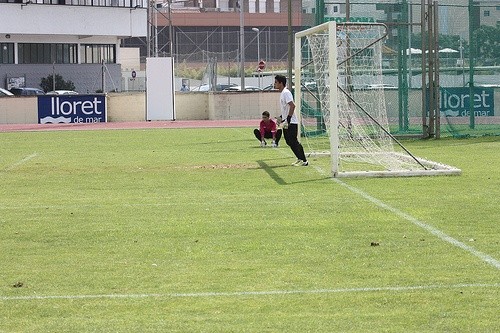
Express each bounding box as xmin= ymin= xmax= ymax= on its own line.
xmin=252 ymin=28 xmax=260 ymax=88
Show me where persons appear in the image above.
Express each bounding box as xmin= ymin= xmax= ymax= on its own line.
xmin=180 ymin=82 xmax=189 ymax=91
xmin=273 ymin=74 xmax=308 ymax=167
xmin=257 ymin=57 xmax=267 ymax=72
xmin=252 ymin=111 xmax=283 ymax=148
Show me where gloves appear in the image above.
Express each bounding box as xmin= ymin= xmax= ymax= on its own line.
xmin=275 ymin=114 xmax=292 ymax=129
xmin=260 ymin=138 xmax=277 ymax=148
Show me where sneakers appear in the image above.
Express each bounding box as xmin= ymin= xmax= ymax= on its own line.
xmin=291 ymin=158 xmax=309 ymax=166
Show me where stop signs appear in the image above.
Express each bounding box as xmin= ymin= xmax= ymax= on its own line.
xmin=259 ymin=61 xmax=265 ymax=70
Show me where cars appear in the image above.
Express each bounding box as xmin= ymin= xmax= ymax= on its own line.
xmin=0 ymin=88 xmax=80 ymax=96
xmin=192 ymin=80 xmax=396 ymax=89
